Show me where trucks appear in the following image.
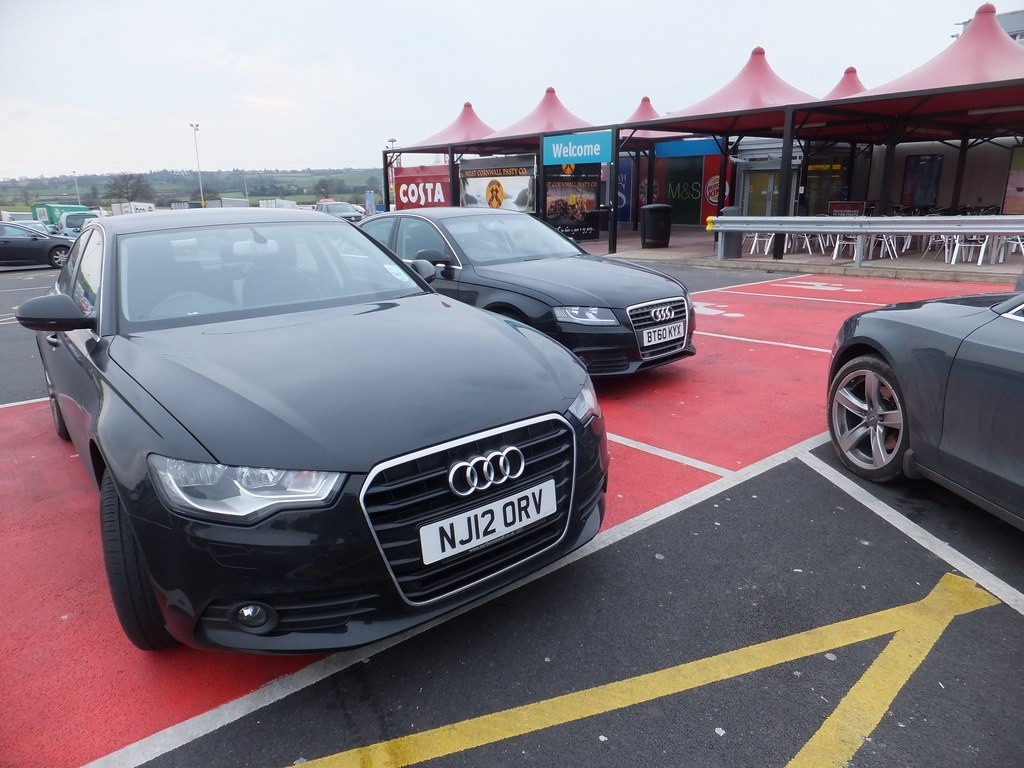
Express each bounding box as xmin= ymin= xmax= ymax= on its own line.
xmin=0 ymin=198 xmax=298 ymax=221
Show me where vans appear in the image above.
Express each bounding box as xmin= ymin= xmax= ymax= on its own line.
xmin=57 ymin=211 xmax=99 ymax=238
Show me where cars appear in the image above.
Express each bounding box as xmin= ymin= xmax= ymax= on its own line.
xmin=0 ymin=221 xmax=76 ymax=268
xmin=827 ymin=289 xmax=1024 ymax=531
xmin=14 ymin=207 xmax=610 ymax=654
xmin=354 ymin=207 xmax=696 ymax=389
xmin=311 ymin=199 xmax=366 ymax=225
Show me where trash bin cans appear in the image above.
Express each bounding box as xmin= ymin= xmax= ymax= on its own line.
xmin=641 ymin=204 xmax=672 ymax=249
xmin=718 ymin=206 xmax=743 ymax=258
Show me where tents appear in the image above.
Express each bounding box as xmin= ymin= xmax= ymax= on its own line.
xmin=383 ymin=2 xmax=1024 ymax=254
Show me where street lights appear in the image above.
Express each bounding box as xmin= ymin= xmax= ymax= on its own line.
xmin=386 ymin=138 xmax=399 ymax=204
xmin=73 ymin=171 xmax=81 ymax=205
xmin=190 ymin=124 xmax=205 ymax=208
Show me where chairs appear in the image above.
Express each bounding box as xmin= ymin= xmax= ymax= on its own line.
xmin=740 ymin=204 xmax=1023 ymax=264
xmin=410 ymin=221 xmax=445 ymax=263
